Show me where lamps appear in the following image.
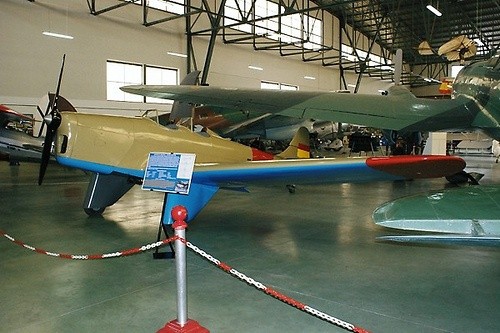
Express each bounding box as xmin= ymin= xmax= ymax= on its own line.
xmin=347 ymin=83 xmax=356 ymax=87
xmin=304 ymin=76 xmax=315 ymax=80
xmin=42 ymin=31 xmax=74 ymax=40
xmin=426 ymin=3 xmax=443 ymax=16
xmin=167 ymin=52 xmax=188 ymax=58
xmin=248 ymin=65 xmax=263 ymax=71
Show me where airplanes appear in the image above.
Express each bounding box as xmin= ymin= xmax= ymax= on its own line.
xmin=37 ymin=50 xmax=467 ymax=232
xmin=0 ymin=104 xmax=57 ymax=161
xmin=117 ymin=57 xmax=500 ymax=243
